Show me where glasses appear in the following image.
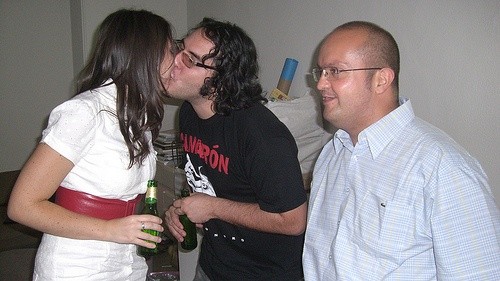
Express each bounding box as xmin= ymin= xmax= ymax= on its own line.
xmin=311 ymin=66 xmax=383 ymax=82
xmin=173 ymin=39 xmax=218 ymax=71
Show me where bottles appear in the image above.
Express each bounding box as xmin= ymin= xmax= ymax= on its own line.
xmin=137 ymin=180 xmax=160 ymax=256
xmin=179 ymin=189 xmax=197 ymax=250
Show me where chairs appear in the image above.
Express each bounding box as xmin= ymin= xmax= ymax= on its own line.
xmin=0 ymin=170 xmax=56 ymax=281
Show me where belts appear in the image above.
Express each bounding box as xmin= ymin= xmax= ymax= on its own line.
xmin=54 ymin=186 xmax=146 ymax=221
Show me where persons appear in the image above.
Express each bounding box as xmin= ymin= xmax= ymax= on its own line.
xmin=162 ymin=17 xmax=308 ymax=281
xmin=8 ymin=7 xmax=166 ymax=281
xmin=302 ymin=21 xmax=500 ymax=281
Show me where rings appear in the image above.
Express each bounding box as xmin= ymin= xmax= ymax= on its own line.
xmin=141 ymin=222 xmax=145 ymax=230
